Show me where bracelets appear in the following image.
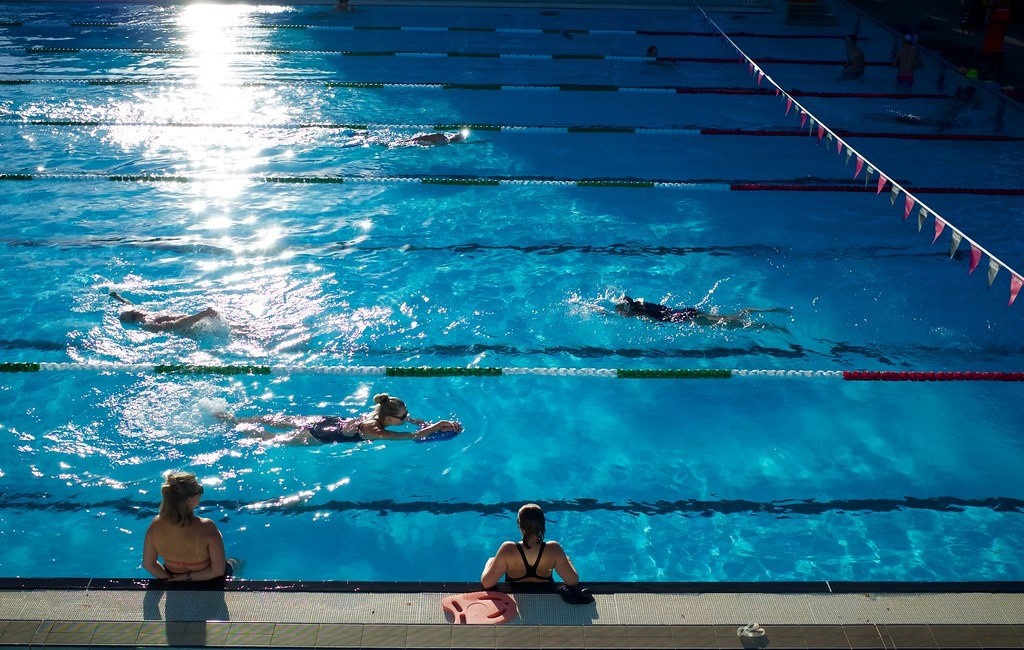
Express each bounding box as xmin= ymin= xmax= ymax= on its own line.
xmin=185 ymin=572 xmax=191 ymax=582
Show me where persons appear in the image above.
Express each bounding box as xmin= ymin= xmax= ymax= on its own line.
xmin=142 ymin=472 xmax=242 ymax=582
xmin=580 ymin=296 xmax=699 ymax=324
xmin=333 ymin=0 xmax=924 ymax=85
xmin=306 ymin=394 xmax=461 ymax=447
xmin=396 ymin=132 xmax=491 ymax=147
xmin=481 ymin=504 xmax=578 ymax=588
xmin=109 ymin=291 xmax=217 ymax=336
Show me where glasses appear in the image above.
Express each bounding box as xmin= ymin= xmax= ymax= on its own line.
xmin=192 ymin=486 xmax=204 ymax=497
xmin=132 ymin=314 xmax=136 ymax=321
xmin=391 ymin=410 xmax=408 ymax=421
